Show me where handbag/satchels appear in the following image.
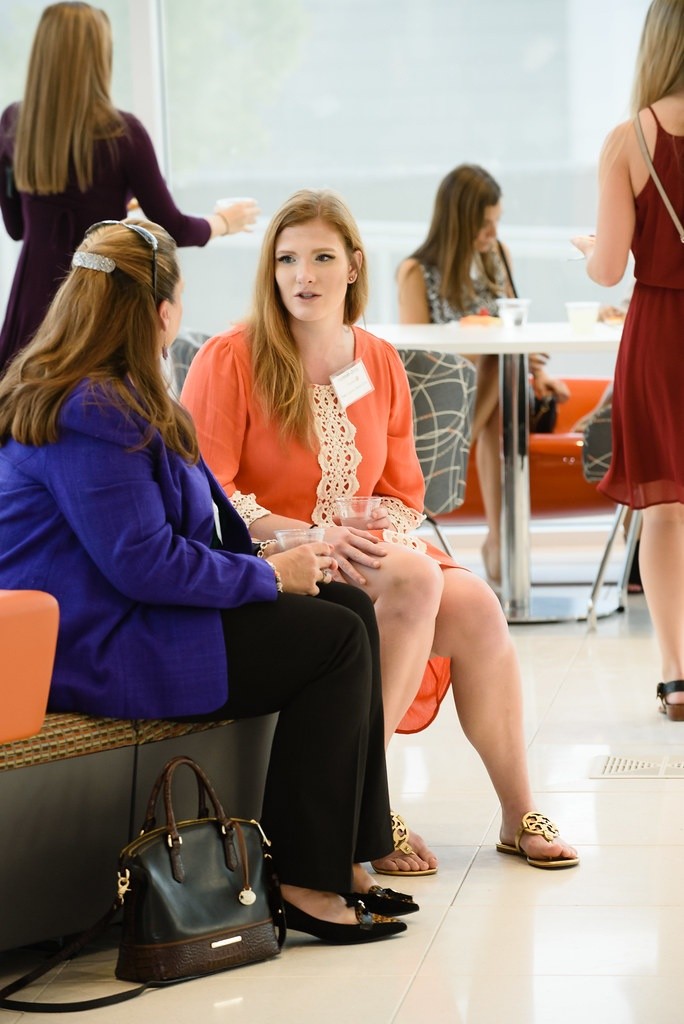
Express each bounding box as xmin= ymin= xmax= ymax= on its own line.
xmin=108 ymin=754 xmax=280 ymax=984
xmin=529 ymin=379 xmax=554 ymax=431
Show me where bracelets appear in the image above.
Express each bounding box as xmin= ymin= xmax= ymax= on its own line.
xmin=256 ymin=539 xmax=278 ymax=558
xmin=309 ymin=524 xmax=318 ymax=529
xmin=215 ymin=212 xmax=229 ymax=236
xmin=265 ymin=559 xmax=283 ymax=593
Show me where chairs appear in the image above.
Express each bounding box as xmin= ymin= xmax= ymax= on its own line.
xmin=582 ymin=402 xmax=642 ymax=624
xmin=169 ymin=330 xmax=214 ymax=402
xmin=392 ymin=349 xmax=478 ymax=560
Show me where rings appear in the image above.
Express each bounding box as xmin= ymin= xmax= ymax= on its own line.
xmin=321 ymin=568 xmax=327 ymax=582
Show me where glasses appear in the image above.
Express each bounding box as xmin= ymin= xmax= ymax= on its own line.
xmin=84 ymin=219 xmax=157 ymax=306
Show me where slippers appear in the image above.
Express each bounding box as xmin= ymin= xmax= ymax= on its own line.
xmin=497 ymin=811 xmax=579 ymax=868
xmin=368 ymin=808 xmax=439 ymax=875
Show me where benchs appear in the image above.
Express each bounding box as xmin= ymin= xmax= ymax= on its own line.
xmin=0 ymin=591 xmax=279 ymax=953
xmin=433 ymin=374 xmax=625 ymax=586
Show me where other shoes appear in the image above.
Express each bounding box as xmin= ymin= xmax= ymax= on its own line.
xmin=622 ymin=540 xmax=643 ymax=594
xmin=482 ymin=543 xmax=504 ymax=595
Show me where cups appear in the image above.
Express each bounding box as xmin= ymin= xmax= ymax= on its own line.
xmin=273 ymin=529 xmax=324 ymax=552
xmin=216 ymin=197 xmax=249 ymax=209
xmin=497 ymin=298 xmax=529 ymax=325
xmin=335 ymin=498 xmax=381 ymax=529
xmin=564 ymin=302 xmax=600 ymax=325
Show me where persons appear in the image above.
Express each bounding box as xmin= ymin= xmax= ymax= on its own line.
xmin=0 ymin=219 xmax=419 ymax=946
xmin=397 ymin=162 xmax=570 ymax=591
xmin=177 ymin=189 xmax=580 ymax=877
xmin=0 ymin=0 xmax=260 ymax=381
xmin=571 ymin=0 xmax=684 ymax=722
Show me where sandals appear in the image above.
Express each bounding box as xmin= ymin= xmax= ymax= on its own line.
xmin=656 ymin=680 xmax=684 ymax=721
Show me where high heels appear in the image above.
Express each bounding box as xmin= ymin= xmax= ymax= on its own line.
xmin=264 ymin=877 xmax=407 ymax=944
xmin=347 ymin=884 xmax=419 ymax=917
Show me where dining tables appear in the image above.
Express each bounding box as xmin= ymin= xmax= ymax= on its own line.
xmin=356 ymin=319 xmax=626 ymax=624
